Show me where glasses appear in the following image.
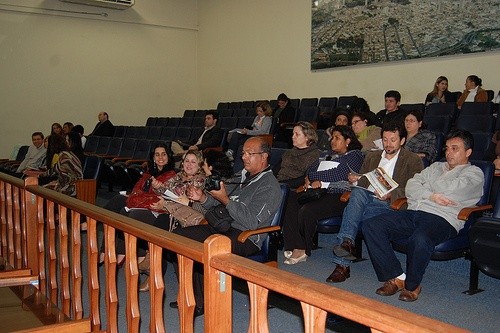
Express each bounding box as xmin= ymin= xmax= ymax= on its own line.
xmin=240 ymin=152 xmax=261 ymax=156
xmin=352 ymin=120 xmax=362 ymax=124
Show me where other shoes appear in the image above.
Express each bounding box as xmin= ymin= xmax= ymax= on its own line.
xmin=194 ymin=301 xmax=204 ymax=317
xmin=139 ymin=276 xmax=150 ymax=292
xmin=138 ymin=250 xmax=150 ymax=275
xmin=116 ymin=255 xmax=126 ymax=269
xmin=284 ymin=251 xmax=307 ymax=265
xmin=169 ymin=301 xmax=179 ymax=308
xmin=98 ymin=253 xmax=104 ymax=267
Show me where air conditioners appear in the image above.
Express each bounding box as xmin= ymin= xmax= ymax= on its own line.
xmin=58 ymin=0 xmax=135 ymax=9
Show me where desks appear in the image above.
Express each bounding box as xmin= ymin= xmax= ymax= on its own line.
xmin=0 ymin=269 xmax=91 ymax=333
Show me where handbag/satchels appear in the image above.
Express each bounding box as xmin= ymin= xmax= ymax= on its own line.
xmin=297 ymin=188 xmax=327 ymax=204
xmin=165 ymin=202 xmax=203 ymax=232
xmin=204 ymin=205 xmax=232 ymax=232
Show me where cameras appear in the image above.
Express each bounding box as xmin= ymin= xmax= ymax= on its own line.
xmin=204 ymin=178 xmax=222 ymax=190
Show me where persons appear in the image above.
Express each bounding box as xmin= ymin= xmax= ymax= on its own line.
xmin=179 ymin=149 xmax=242 ymax=226
xmin=127 ymin=149 xmax=207 ymax=292
xmin=362 ymin=127 xmax=485 ymax=302
xmin=39 ymin=133 xmax=83 ymax=224
xmin=375 ymin=91 xmax=405 ymax=129
xmin=98 ymin=142 xmax=176 ymax=268
xmin=326 ymin=112 xmax=436 ymax=160
xmin=486 ymin=130 xmax=500 ymax=217
xmin=227 ymin=102 xmax=272 ymax=149
xmin=270 ymin=93 xmax=296 ymax=135
xmin=171 ymin=111 xmax=226 ymax=154
xmin=277 ymin=122 xmax=365 ymax=265
xmin=88 ymin=112 xmax=113 ymax=136
xmin=170 ymin=137 xmax=282 ymax=317
xmin=326 ymin=118 xmax=424 ymax=283
xmin=425 ymin=75 xmax=488 ymax=109
xmin=51 ymin=122 xmax=87 ymax=149
xmin=11 ymin=132 xmax=49 ymax=180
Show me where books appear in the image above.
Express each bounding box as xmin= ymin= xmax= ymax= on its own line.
xmin=346 ymin=163 xmax=399 ymax=198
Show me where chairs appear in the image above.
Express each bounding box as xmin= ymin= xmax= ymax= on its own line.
xmin=1 ymin=95 xmax=500 ymax=298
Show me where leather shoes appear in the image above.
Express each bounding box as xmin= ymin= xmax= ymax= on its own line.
xmin=333 ymin=242 xmax=358 ymax=261
xmin=399 ymin=286 xmax=421 ymax=302
xmin=375 ymin=278 xmax=405 ymax=297
xmin=326 ymin=264 xmax=351 ymax=283
